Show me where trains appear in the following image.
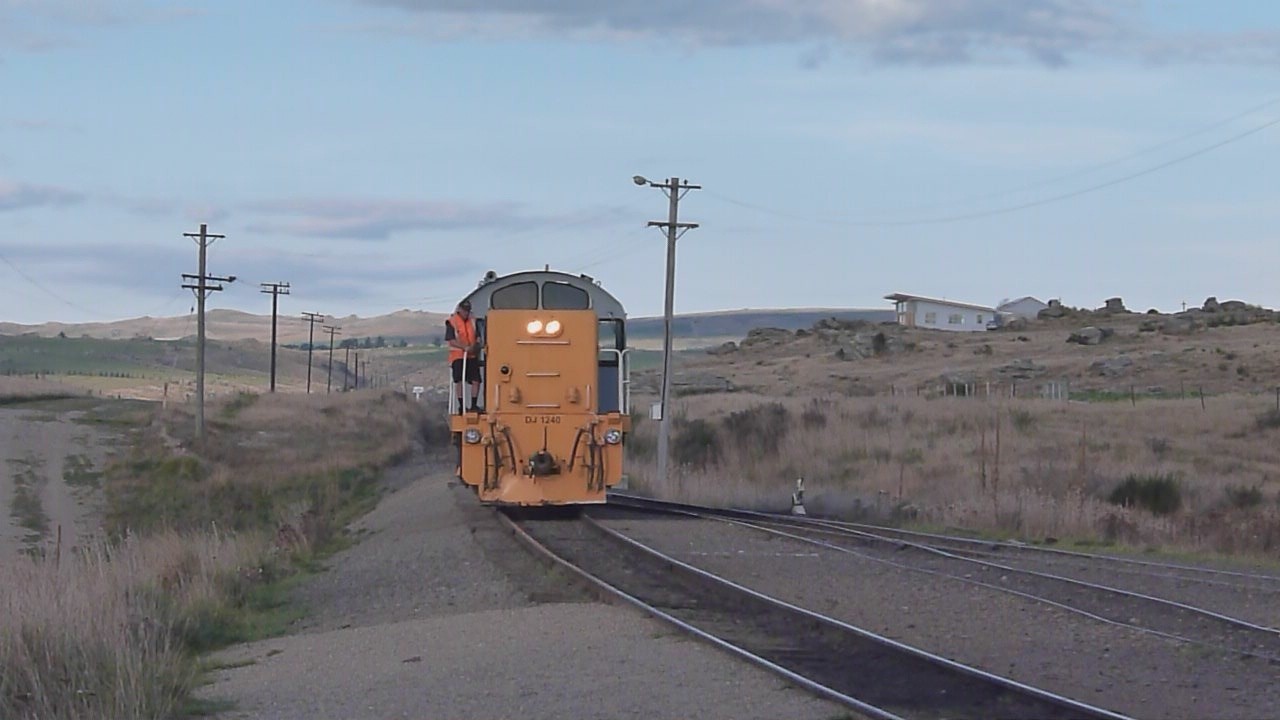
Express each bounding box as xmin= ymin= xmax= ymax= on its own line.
xmin=444 ymin=262 xmax=632 ymax=517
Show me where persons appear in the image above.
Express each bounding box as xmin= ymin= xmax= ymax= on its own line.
xmin=445 ymin=300 xmax=484 ymax=414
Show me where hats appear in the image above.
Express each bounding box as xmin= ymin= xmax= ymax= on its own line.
xmin=460 ymin=300 xmax=471 ymax=310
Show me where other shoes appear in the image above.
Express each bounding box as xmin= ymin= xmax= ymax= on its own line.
xmin=471 ymin=407 xmax=479 ymax=410
xmin=459 ymin=408 xmax=462 ymax=415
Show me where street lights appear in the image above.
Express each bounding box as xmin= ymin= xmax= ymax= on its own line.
xmin=634 ymin=175 xmax=680 ymax=479
